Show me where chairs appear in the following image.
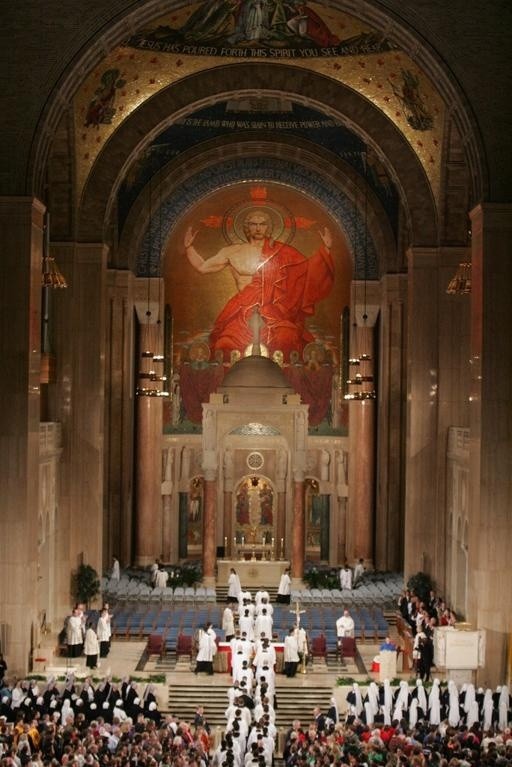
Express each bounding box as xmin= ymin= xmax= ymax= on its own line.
xmin=309 ymin=635 xmax=356 ymax=665
xmin=146 ymin=632 xmax=193 ymax=663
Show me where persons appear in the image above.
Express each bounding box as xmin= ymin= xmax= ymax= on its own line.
xmin=225 ymin=566 xmax=277 ymax=670
xmin=111 ymin=555 xmax=119 ymax=580
xmin=353 ymin=558 xmax=365 ymax=586
xmin=340 ymin=564 xmax=352 ymax=589
xmin=336 ymin=610 xmax=355 ymax=648
xmin=65 ymin=601 xmax=114 ymax=670
xmin=396 ymin=587 xmax=458 ymax=671
xmin=182 ymin=211 xmax=336 ymax=364
xmin=277 ymin=569 xmax=292 ymax=604
xmin=284 ymin=630 xmax=299 ymax=676
xmin=291 ymin=622 xmax=310 ymax=666
xmin=150 ymin=560 xmax=161 ymax=572
xmin=152 ymin=566 xmax=169 ymax=588
xmin=1 ymin=670 xmax=512 ymax=767
